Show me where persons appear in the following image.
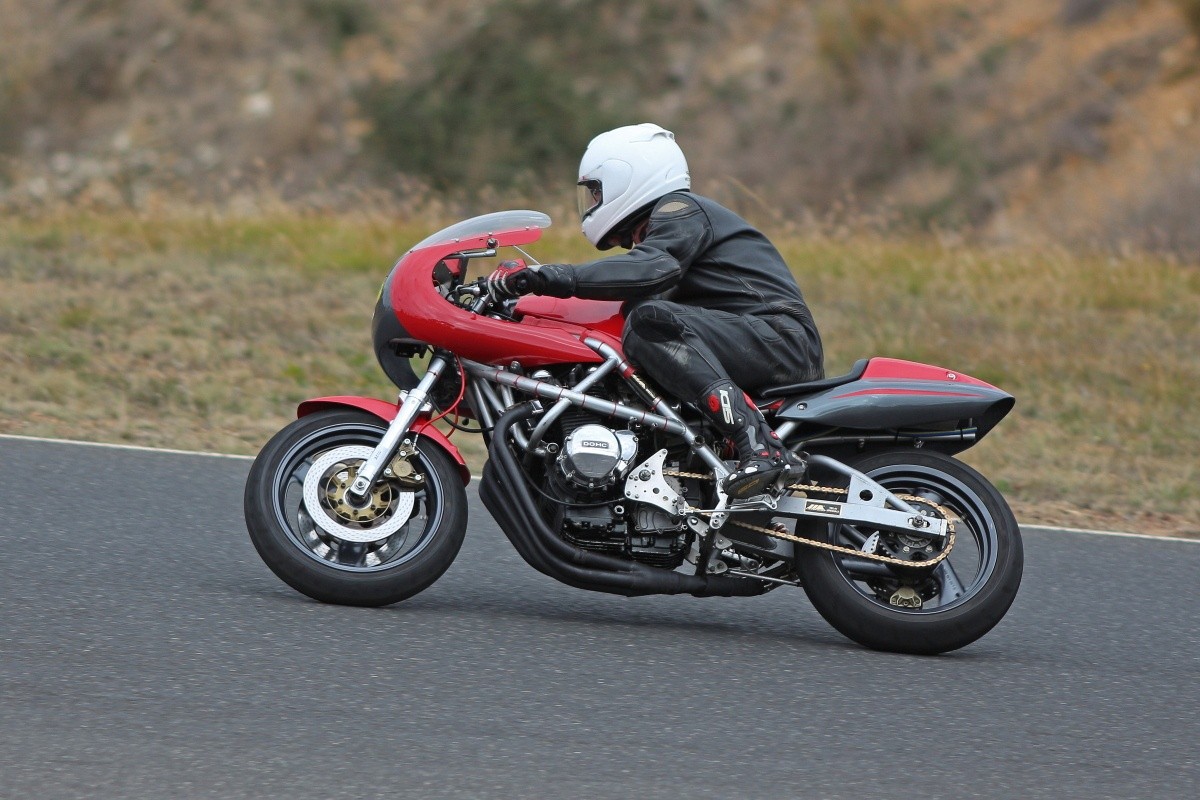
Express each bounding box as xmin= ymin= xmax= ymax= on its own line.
xmin=486 ymin=122 xmax=824 ymax=497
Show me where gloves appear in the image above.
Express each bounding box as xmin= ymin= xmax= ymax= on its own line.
xmin=483 ymin=258 xmax=545 ymax=305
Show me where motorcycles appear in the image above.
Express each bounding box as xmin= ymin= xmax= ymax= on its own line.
xmin=244 ymin=211 xmax=1027 ymax=654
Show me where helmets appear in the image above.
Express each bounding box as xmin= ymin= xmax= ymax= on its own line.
xmin=576 ymin=122 xmax=692 ymax=253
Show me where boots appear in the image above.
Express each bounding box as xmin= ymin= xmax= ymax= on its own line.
xmin=693 ymin=376 xmax=807 ymax=500
